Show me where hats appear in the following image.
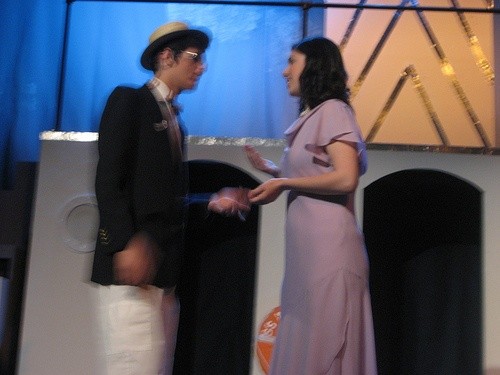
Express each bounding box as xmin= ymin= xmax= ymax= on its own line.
xmin=140 ymin=23 xmax=211 ymax=72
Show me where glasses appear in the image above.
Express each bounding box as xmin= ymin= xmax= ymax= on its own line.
xmin=170 ymin=47 xmax=203 ymax=63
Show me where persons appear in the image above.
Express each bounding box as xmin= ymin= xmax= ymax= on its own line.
xmin=88 ymin=21 xmax=248 ymax=375
xmin=244 ymin=36 xmax=376 ymax=375
